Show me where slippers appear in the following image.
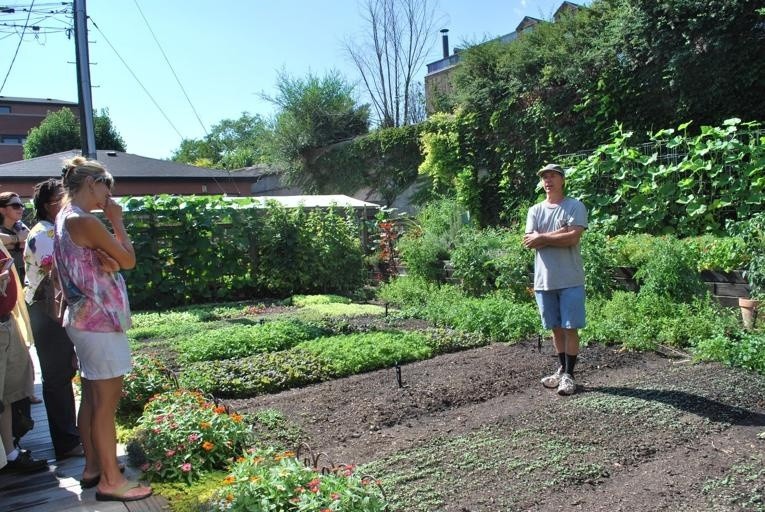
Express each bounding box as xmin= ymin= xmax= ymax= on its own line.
xmin=80 ymin=467 xmax=153 ymax=501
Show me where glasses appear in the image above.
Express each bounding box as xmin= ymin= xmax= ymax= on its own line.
xmin=5 ymin=203 xmax=24 ymax=210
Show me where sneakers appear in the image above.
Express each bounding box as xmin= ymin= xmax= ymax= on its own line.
xmin=541 ymin=369 xmax=577 ymax=396
xmin=30 ymin=397 xmax=42 ymax=403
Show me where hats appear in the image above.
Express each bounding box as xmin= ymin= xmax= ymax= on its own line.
xmin=536 ymin=164 xmax=565 ymax=176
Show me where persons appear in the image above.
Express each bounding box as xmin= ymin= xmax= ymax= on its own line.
xmin=0 ymin=155 xmax=154 ymax=502
xmin=522 ymin=163 xmax=589 ymax=396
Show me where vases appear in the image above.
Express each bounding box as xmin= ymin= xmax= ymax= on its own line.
xmin=738 ymin=297 xmax=762 ymax=332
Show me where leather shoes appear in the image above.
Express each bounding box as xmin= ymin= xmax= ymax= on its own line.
xmin=5 ymin=451 xmax=48 ymax=472
